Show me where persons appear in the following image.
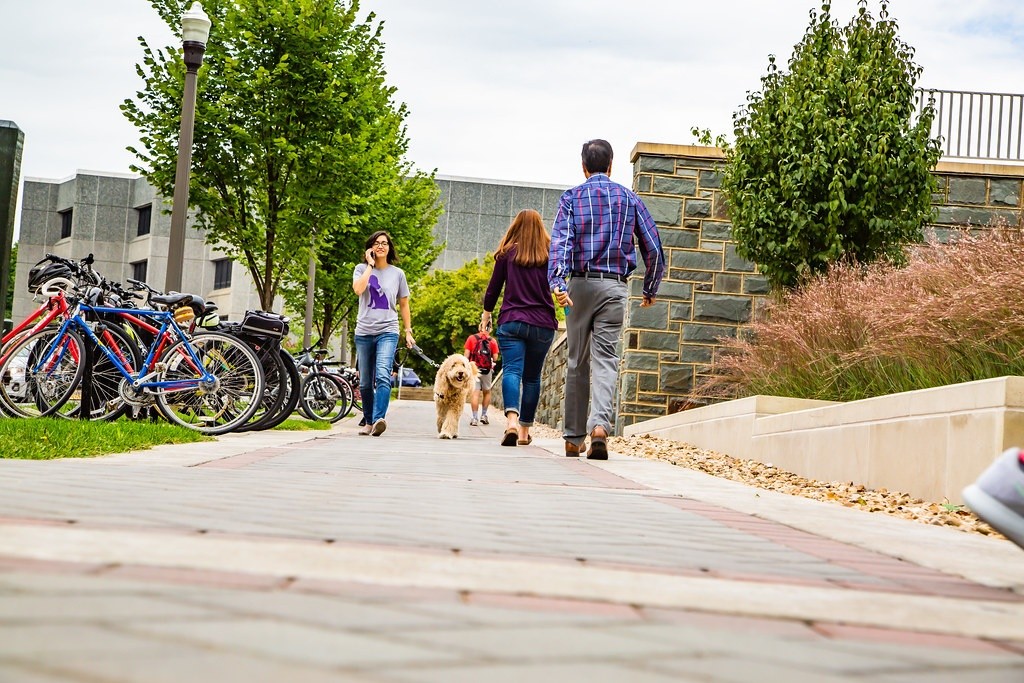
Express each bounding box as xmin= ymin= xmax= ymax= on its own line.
xmin=478 ymin=209 xmax=566 ymax=446
xmin=547 ymin=140 xmax=666 ymax=460
xmin=351 ymin=231 xmax=416 ymax=437
xmin=464 ymin=319 xmax=499 ymax=426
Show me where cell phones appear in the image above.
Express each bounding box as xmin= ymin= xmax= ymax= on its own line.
xmin=559 ymin=285 xmax=570 ymax=316
xmin=371 ymin=251 xmax=375 ymax=259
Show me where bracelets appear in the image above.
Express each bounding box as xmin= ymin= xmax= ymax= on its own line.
xmin=368 ymin=263 xmax=374 ymax=268
xmin=481 ymin=314 xmax=492 ymax=319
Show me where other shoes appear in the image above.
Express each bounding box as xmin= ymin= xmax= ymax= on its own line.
xmin=359 ymin=428 xmax=371 ymax=435
xmin=371 ymin=418 xmax=386 ymax=436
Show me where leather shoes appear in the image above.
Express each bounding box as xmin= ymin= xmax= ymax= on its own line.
xmin=566 ymin=440 xmax=586 ymax=457
xmin=587 ymin=428 xmax=608 ymax=460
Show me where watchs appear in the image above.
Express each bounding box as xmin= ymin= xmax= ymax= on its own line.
xmin=404 ymin=328 xmax=413 ymax=333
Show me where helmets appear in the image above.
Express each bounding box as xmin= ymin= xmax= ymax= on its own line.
xmin=27 ymin=262 xmax=72 ymax=295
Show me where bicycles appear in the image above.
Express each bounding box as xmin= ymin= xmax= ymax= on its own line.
xmin=0 ymin=253 xmax=374 ymax=436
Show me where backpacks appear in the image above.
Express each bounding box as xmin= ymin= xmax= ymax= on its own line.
xmin=470 ymin=334 xmax=492 ymax=374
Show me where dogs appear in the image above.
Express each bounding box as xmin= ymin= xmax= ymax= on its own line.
xmin=433 ymin=354 xmax=482 ymax=439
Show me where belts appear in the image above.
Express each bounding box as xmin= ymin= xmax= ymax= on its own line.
xmin=571 ymin=271 xmax=627 ymax=283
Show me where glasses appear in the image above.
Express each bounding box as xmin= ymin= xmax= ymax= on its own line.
xmin=372 ymin=241 xmax=389 ymax=248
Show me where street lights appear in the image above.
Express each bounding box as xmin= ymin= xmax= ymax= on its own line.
xmin=164 ymin=2 xmax=212 ymax=296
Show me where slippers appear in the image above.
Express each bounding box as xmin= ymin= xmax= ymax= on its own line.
xmin=500 ymin=428 xmax=518 ymax=445
xmin=518 ymin=434 xmax=531 ymax=445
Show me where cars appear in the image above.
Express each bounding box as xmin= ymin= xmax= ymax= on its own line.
xmin=389 ymin=368 xmax=422 ymax=388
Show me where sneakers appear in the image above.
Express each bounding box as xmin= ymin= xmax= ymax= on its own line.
xmin=480 ymin=415 xmax=489 ymax=424
xmin=470 ymin=418 xmax=478 ymax=425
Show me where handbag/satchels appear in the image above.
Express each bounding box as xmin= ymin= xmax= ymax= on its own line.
xmin=242 ymin=310 xmax=289 ymax=340
